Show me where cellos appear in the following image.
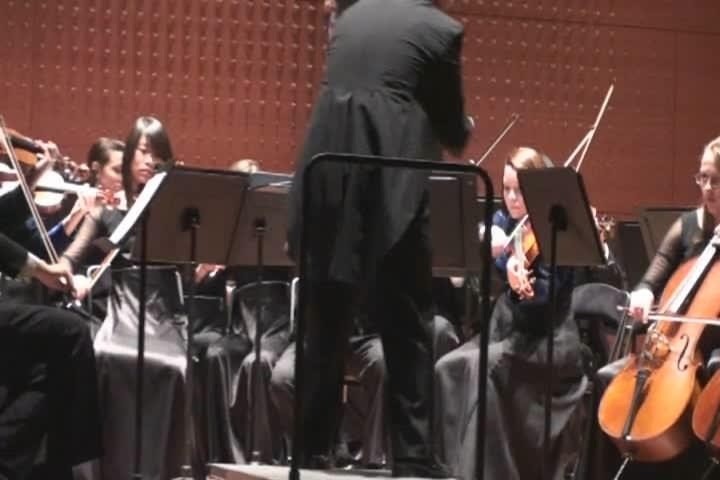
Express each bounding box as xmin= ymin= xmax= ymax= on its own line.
xmin=598 ymin=223 xmax=720 ymax=462
xmin=692 ymin=367 xmax=720 ymax=447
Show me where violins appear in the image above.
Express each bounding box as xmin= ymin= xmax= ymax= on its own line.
xmin=0 ymin=127 xmax=120 ymax=213
xmin=514 ymin=223 xmax=541 ymax=300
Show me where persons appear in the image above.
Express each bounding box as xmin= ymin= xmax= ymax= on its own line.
xmin=289 ymin=1 xmax=476 ymax=480
xmin=0 ymin=116 xmax=456 ymax=479
xmin=433 ymin=134 xmax=720 ymax=480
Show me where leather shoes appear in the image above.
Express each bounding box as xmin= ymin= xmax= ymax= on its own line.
xmin=305 ymin=454 xmax=334 ymax=469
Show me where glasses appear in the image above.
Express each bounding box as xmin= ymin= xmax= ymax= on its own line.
xmin=695 ymin=172 xmax=719 ymax=190
xmin=391 ymin=461 xmax=450 ymax=478
